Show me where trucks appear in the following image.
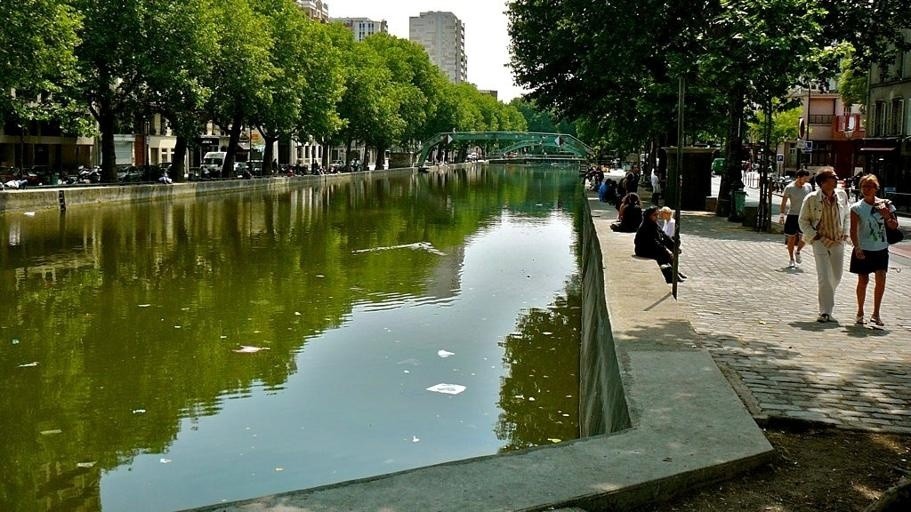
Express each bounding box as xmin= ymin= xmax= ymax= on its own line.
xmin=199 ymin=151 xmax=227 ymax=174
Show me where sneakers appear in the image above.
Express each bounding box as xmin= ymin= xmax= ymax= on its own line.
xmin=789 ymin=258 xmax=796 ymax=267
xmin=817 ymin=313 xmax=837 ymax=323
xmin=871 ymin=315 xmax=885 ymax=326
xmin=677 ymin=272 xmax=689 ymax=282
xmin=796 ymin=251 xmax=802 ymax=263
xmin=855 ymin=314 xmax=868 ymax=324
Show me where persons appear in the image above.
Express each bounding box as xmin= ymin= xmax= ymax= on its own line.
xmin=741 ymin=158 xmax=898 ymax=326
xmin=581 ymin=162 xmax=687 ymax=284
xmin=158 ymin=168 xmax=173 ymax=184
xmin=272 ymin=159 xmax=342 ymax=177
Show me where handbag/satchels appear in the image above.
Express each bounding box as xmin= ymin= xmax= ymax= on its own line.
xmin=885 ymin=218 xmax=904 ymax=244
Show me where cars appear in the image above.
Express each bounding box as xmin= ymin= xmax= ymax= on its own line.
xmin=1 ymin=161 xmax=174 ymax=184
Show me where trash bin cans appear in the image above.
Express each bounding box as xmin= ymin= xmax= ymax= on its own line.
xmin=729 ymin=180 xmax=746 ymax=222
xmin=50 ymin=170 xmax=60 ymax=185
xmin=661 ymin=145 xmax=726 ymax=209
